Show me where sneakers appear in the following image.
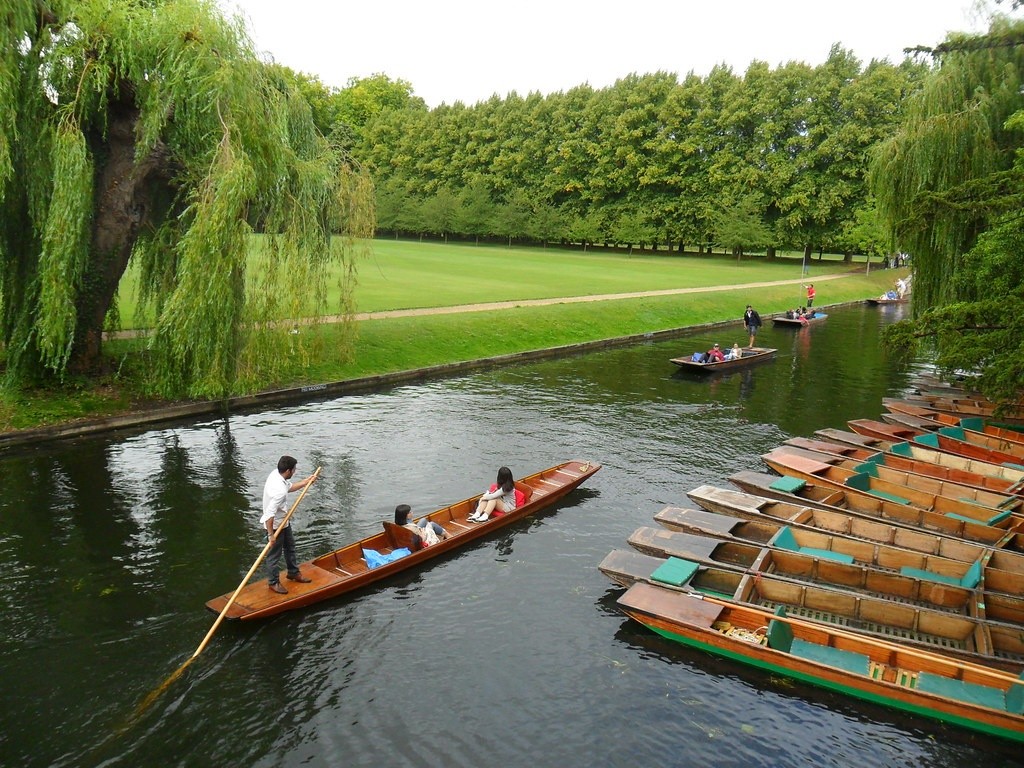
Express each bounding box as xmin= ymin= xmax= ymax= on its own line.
xmin=466 ymin=514 xmax=480 ymax=523
xmin=473 ymin=515 xmax=489 ymax=524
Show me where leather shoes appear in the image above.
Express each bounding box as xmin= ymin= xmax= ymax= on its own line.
xmin=269 ymin=580 xmax=288 ymax=595
xmin=285 ymin=570 xmax=312 ymax=584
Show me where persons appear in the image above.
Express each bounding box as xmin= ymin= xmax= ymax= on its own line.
xmin=728 ymin=343 xmax=742 ymax=360
xmin=395 ymin=504 xmax=454 ymax=548
xmin=698 ymin=344 xmax=725 ymax=363
xmin=881 ymin=250 xmax=909 ymax=300
xmin=787 ymin=307 xmax=817 ymax=321
xmin=259 ymin=455 xmax=317 ymax=594
xmin=743 ymin=304 xmax=762 ymax=349
xmin=800 ymin=282 xmax=815 ymax=307
xmin=466 ymin=467 xmax=516 ymax=524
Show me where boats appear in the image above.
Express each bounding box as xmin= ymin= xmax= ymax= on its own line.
xmin=773 ymin=312 xmax=828 ymax=326
xmin=206 ymin=460 xmax=599 ymax=622
xmin=599 ymin=361 xmax=1024 ymax=768
xmin=671 ymin=346 xmax=777 ymax=370
xmin=866 ymin=298 xmax=911 ymax=304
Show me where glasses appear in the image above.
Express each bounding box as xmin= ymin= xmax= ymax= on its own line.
xmin=714 ymin=345 xmax=719 ymax=348
